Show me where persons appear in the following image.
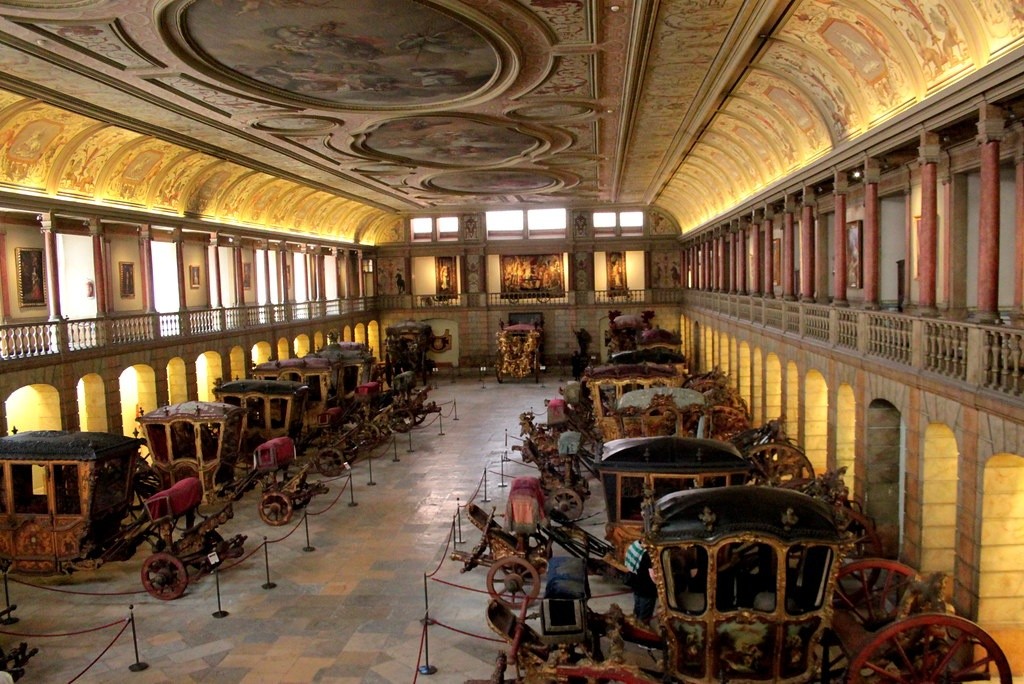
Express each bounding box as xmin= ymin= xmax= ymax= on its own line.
xmin=571 ymin=351 xmax=582 ymax=382
xmin=624 ymin=527 xmax=658 ymax=651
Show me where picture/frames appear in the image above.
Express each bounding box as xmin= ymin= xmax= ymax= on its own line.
xmin=15 ymin=247 xmax=48 ymax=309
xmin=844 ymin=220 xmax=862 ymax=289
xmin=242 ymin=263 xmax=251 ymax=290
xmin=189 ymin=265 xmax=201 ymax=288
xmin=774 ymin=238 xmax=780 ymax=286
xmin=119 ymin=261 xmax=135 ymax=300
xmin=287 ymin=265 xmax=291 ymax=289
